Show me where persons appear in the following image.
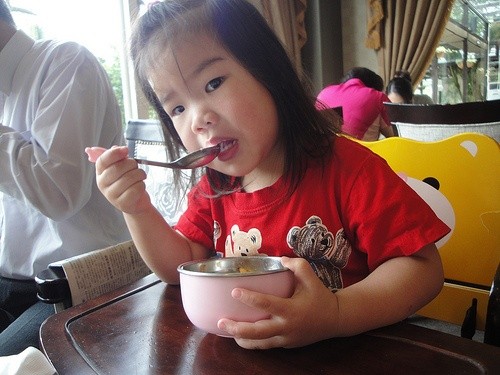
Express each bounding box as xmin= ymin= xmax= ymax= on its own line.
xmin=315 ymin=67 xmax=394 ymax=141
xmin=0 ymin=0 xmax=132 ymax=358
xmin=387 ymin=70 xmax=413 ymax=104
xmin=95 ymin=0 xmax=452 ymax=349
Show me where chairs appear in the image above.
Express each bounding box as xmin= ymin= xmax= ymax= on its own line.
xmin=34 ymin=98 xmax=500 ymax=375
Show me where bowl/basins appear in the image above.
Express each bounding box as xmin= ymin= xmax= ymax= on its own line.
xmin=176 ymin=255 xmax=295 ymax=339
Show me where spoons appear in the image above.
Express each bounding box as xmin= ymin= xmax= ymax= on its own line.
xmin=84 ymin=144 xmax=222 ymax=169
